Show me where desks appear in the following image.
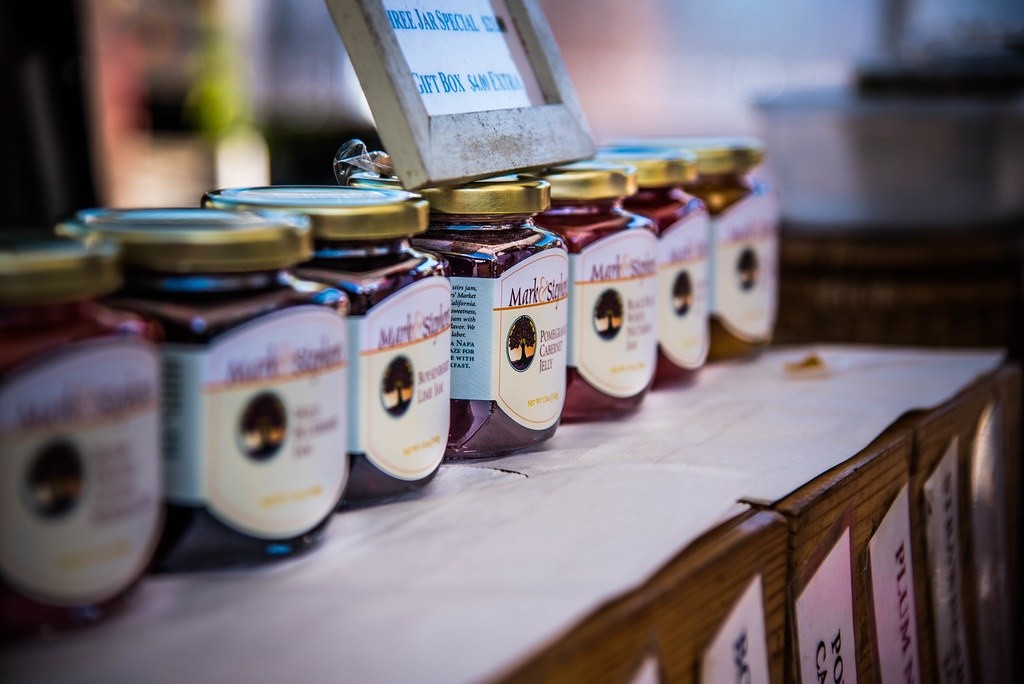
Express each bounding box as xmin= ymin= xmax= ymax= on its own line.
xmin=771 ymin=218 xmax=1024 ymax=365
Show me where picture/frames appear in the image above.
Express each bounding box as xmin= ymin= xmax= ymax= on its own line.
xmin=327 ymin=1 xmax=601 ymax=189
